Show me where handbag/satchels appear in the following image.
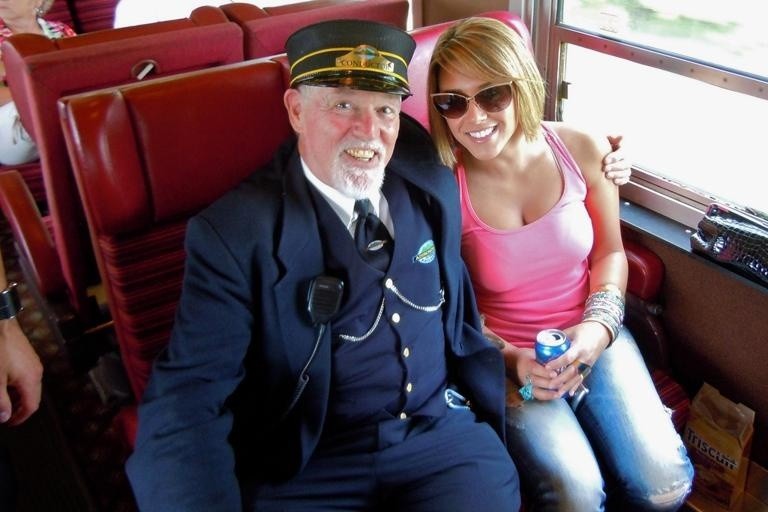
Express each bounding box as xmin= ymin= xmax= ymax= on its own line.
xmin=690 ymin=202 xmax=768 ymax=286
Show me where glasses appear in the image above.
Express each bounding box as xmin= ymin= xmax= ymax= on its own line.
xmin=430 ymin=80 xmax=514 ymax=120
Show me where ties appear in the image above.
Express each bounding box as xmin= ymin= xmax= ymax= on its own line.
xmin=354 ymin=198 xmax=395 ymax=273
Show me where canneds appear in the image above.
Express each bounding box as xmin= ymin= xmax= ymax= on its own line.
xmin=535 ymin=328 xmax=570 ymax=398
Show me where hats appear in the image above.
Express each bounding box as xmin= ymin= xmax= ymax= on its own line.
xmin=284 ymin=19 xmax=417 ymax=101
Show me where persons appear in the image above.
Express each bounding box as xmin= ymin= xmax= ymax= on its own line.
xmin=426 ymin=16 xmax=697 ymax=511
xmin=125 ymin=17 xmax=632 ymax=512
xmin=0 ymin=256 xmax=47 ymax=430
xmin=113 ymin=0 xmax=225 ymax=29
xmin=0 ymin=1 xmax=77 ymax=167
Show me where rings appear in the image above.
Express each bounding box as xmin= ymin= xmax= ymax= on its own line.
xmin=519 ymin=380 xmax=534 ymax=404
xmin=571 ymin=359 xmax=591 ymax=377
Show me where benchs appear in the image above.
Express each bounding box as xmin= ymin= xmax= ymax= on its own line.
xmin=0 ymin=1 xmax=692 ymax=490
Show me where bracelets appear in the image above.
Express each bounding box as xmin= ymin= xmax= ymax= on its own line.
xmin=0 ymin=280 xmax=25 ymax=324
xmin=579 ymin=284 xmax=627 ymax=349
